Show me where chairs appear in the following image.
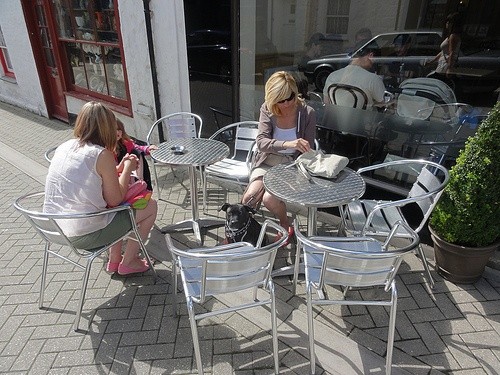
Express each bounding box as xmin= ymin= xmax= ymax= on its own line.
xmin=395 ymin=102 xmax=473 ymax=183
xmin=13 ymin=82 xmax=451 ymax=375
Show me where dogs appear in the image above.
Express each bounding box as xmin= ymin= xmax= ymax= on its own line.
xmin=217 ymin=202 xmax=269 ymax=248
xmin=374 ymin=127 xmax=399 ymax=146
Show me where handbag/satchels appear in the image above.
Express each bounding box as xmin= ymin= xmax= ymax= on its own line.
xmin=122 ymin=176 xmax=147 ymax=201
xmin=284 ymin=148 xmax=350 ymax=180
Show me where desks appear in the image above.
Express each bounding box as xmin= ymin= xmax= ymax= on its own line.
xmin=261 ymin=161 xmax=366 ymax=300
xmin=149 ymin=138 xmax=230 ymax=247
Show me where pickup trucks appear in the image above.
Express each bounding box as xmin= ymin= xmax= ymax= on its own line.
xmin=305 ymin=31 xmax=500 ymax=99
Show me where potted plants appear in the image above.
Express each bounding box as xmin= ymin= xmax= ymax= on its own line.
xmin=427 ymin=98 xmax=500 ymax=286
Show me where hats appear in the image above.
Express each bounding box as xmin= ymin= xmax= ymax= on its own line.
xmin=305 ymin=33 xmax=325 ymax=45
xmin=393 ymin=34 xmax=411 ymax=44
xmin=348 ymin=40 xmax=378 ymax=55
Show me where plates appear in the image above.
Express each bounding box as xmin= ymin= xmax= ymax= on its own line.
xmin=169 ymin=146 xmax=185 ymax=153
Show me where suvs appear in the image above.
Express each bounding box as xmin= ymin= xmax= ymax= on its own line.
xmin=186 ymin=28 xmax=285 ymax=85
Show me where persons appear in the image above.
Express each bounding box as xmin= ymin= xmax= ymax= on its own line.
xmin=325 ymin=39 xmax=386 ymax=134
xmin=242 ymin=71 xmax=315 ymax=246
xmin=387 ymin=34 xmax=420 ymax=79
xmin=357 ymin=29 xmax=382 ymax=74
xmin=112 ymin=117 xmax=156 ymax=190
xmin=299 ymin=33 xmax=325 ymax=82
xmin=42 ymin=101 xmax=158 ymax=276
xmin=425 ymin=12 xmax=463 ymax=82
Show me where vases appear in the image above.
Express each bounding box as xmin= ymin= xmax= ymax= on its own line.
xmin=75 ymin=17 xmax=86 ymax=27
xmin=96 ymin=12 xmax=108 ymax=30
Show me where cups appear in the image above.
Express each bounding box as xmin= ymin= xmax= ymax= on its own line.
xmin=75 ymin=17 xmax=86 ymax=28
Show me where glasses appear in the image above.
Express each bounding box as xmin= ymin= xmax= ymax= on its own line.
xmin=278 ymin=92 xmax=295 ymax=103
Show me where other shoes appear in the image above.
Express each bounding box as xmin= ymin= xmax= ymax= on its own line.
xmin=107 ymin=262 xmax=119 ymax=272
xmin=118 ymin=260 xmax=154 ymax=274
xmin=274 ymin=227 xmax=293 ymax=249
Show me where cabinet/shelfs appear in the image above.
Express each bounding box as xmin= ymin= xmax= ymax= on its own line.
xmin=48 ymin=0 xmax=127 ymax=102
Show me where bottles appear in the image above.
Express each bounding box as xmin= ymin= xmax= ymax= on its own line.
xmin=109 ymin=0 xmax=114 ymax=8
